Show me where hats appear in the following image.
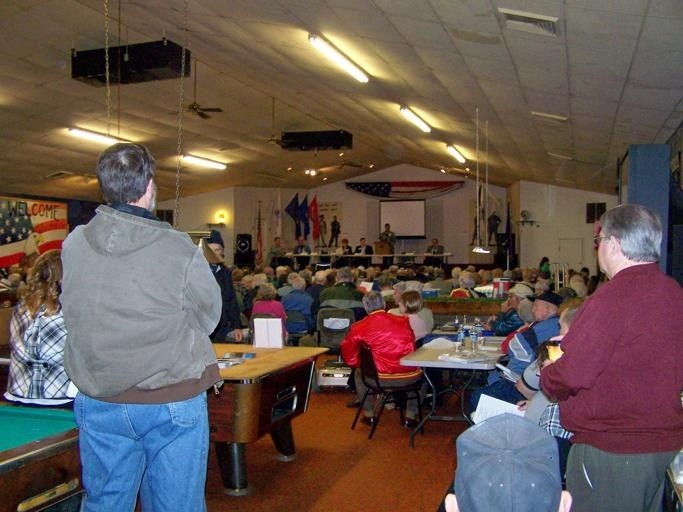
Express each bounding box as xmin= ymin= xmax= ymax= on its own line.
xmin=455 ymin=414 xmax=562 ymax=512
xmin=525 ymin=290 xmax=564 ymax=309
xmin=207 ymin=229 xmax=224 ymax=248
xmin=509 ymin=284 xmax=533 ymax=299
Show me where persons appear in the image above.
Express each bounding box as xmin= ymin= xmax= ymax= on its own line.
xmin=234 ymin=264 xmax=359 ymax=344
xmin=60 ymin=142 xmax=222 ymax=511
xmin=203 ymin=229 xmax=243 ymax=344
xmin=424 ymin=237 xmax=444 ymax=265
xmin=0 ymin=250 xmax=40 ymax=307
xmin=359 ymin=258 xmax=610 ymax=335
xmin=379 ymin=223 xmax=396 ymax=269
xmin=539 ymin=201 xmax=683 ymax=512
xmin=337 ymin=239 xmax=353 ymax=265
xmin=270 ymin=237 xmax=286 ymax=269
xmin=316 ymin=214 xmax=327 ymax=248
xmin=341 ymin=291 xmax=424 ymax=434
xmin=293 ymin=236 xmax=311 ymax=269
xmin=443 ymin=412 xmax=573 ymax=512
xmin=353 ymin=238 xmax=373 ymax=267
xmin=3 ymin=250 xmax=79 ymax=410
xmin=516 ymin=296 xmax=586 ymax=423
xmin=328 ymin=215 xmax=341 ymax=247
xmin=463 ymin=291 xmax=564 ymax=426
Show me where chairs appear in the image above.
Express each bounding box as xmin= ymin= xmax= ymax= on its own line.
xmin=250 ymin=314 xmax=285 ymax=351
xmin=319 ymin=309 xmax=348 ymax=355
xmin=351 ymin=343 xmax=425 ymax=439
xmin=316 ymin=255 xmax=331 ymax=271
xmin=285 ymin=310 xmax=307 ymax=344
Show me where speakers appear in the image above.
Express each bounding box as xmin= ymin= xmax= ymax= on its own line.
xmin=586 ymin=202 xmax=606 ymax=224
xmin=235 ymin=234 xmax=252 ymax=268
xmin=495 ymin=233 xmax=516 ymax=270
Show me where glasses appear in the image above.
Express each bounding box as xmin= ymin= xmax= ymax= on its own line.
xmin=591 ymin=234 xmax=609 ymax=245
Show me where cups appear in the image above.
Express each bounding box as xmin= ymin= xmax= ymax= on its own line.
xmin=478 ymin=336 xmax=486 ymax=345
xmin=454 ymin=341 xmax=463 ymax=353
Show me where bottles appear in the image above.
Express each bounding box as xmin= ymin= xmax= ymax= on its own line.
xmin=469 ymin=324 xmax=477 ymax=354
xmin=457 ymin=325 xmax=467 ymax=349
xmin=552 ymin=262 xmax=561 ymax=295
xmin=454 ymin=314 xmax=461 ymax=329
xmin=463 ymin=313 xmax=469 ymax=327
xmin=562 ymin=260 xmax=569 ymax=292
xmin=473 ymin=316 xmax=484 ymax=353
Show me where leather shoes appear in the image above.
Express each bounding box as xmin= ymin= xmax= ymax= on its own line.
xmin=403 ymin=417 xmax=418 ymax=430
xmin=361 ymin=416 xmax=375 ymax=426
xmin=425 ymin=397 xmax=433 ymax=410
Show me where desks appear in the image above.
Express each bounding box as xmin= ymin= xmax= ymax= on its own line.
xmin=0 ymin=402 xmax=94 ymax=511
xmin=399 ymin=335 xmax=510 ymax=448
xmin=201 ymin=341 xmax=331 ymax=469
xmin=431 ymin=318 xmax=491 ymax=336
xmin=276 ymin=254 xmax=455 ymax=267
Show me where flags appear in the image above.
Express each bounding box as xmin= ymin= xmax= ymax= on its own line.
xmin=299 ymin=193 xmax=310 ymax=240
xmin=308 ymin=194 xmax=320 ymax=240
xmin=286 ymin=192 xmax=302 ymax=241
xmin=343 ymin=180 xmax=464 ymax=201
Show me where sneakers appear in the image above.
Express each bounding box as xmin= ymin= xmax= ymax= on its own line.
xmin=346 ymin=400 xmax=360 ymax=407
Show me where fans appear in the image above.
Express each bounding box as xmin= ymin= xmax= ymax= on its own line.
xmin=261 ymin=96 xmax=292 ymax=150
xmin=169 ymin=54 xmax=224 ymax=120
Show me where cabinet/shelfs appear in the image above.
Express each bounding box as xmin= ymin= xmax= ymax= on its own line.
xmin=617 ymin=143 xmax=668 ymax=272
